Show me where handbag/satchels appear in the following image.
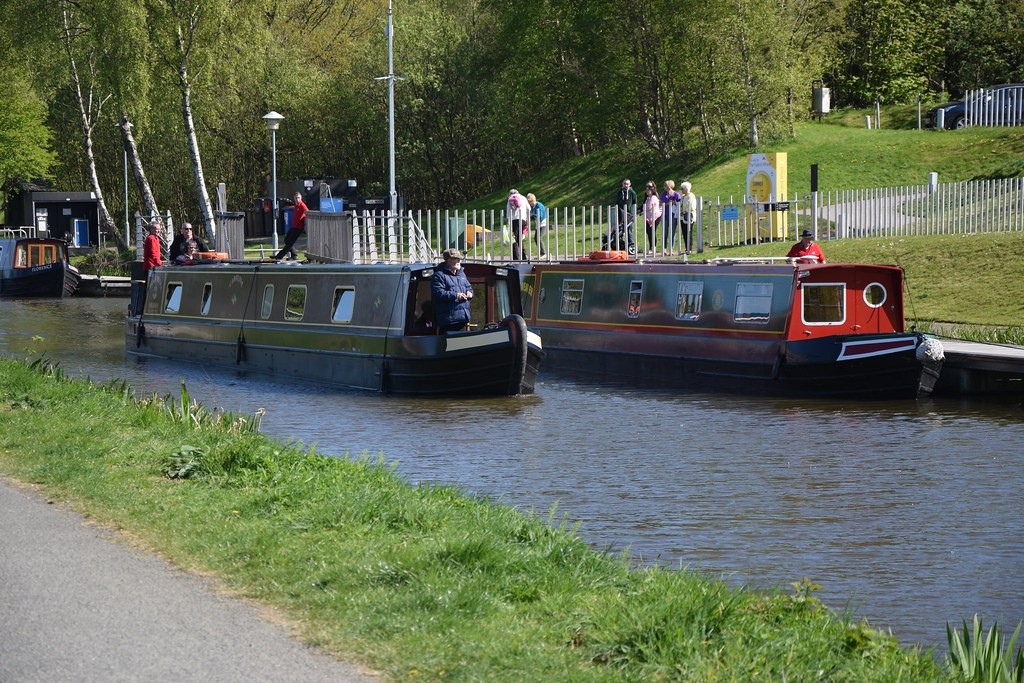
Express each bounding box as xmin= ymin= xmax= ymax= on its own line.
xmin=503 ymin=224 xmax=516 ymax=245
xmin=684 ymin=212 xmax=693 ymax=222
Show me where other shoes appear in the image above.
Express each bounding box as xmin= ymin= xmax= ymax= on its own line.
xmin=533 ymin=253 xmax=547 ymax=259
xmin=270 ymin=256 xmax=281 ymax=259
xmin=286 ymin=256 xmax=298 ymax=260
xmin=648 ymin=249 xmax=654 ymax=255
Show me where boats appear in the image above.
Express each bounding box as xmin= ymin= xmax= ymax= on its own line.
xmin=495 ymin=256 xmax=923 ymax=382
xmin=0 ymin=225 xmax=83 ymax=299
xmin=124 ymin=260 xmax=545 ymax=397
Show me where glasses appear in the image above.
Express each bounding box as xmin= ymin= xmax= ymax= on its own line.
xmin=185 ymin=228 xmax=193 ymax=230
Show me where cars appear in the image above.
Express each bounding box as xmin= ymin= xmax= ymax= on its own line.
xmin=924 ymin=84 xmax=1024 ymax=132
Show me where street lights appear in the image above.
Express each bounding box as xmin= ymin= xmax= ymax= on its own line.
xmin=115 ymin=121 xmax=135 ymax=248
xmin=261 ymin=111 xmax=285 ymax=258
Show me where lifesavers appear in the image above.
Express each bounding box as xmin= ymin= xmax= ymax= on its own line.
xmin=588 ymin=248 xmax=629 ymax=262
xmin=190 ymin=250 xmax=230 ymax=261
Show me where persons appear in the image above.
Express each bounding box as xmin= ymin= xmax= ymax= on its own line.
xmin=784 ymin=230 xmax=826 ymax=265
xmin=616 ymin=179 xmax=697 ymax=256
xmin=268 ymin=193 xmax=309 ymax=259
xmin=505 ymin=188 xmax=548 ymax=260
xmin=414 ymin=248 xmax=475 ymax=335
xmin=171 ymin=223 xmax=211 ymax=266
xmin=141 ymin=221 xmax=164 ymax=317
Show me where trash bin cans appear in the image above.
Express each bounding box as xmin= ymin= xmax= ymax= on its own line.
xmin=283 ymin=206 xmax=296 ymax=234
xmin=441 ymin=217 xmax=467 ymax=251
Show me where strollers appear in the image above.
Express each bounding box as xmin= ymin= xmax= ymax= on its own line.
xmin=602 ymin=219 xmax=634 ymax=255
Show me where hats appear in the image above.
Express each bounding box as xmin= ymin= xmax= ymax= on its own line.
xmin=802 ymin=230 xmax=814 ymax=238
xmin=443 ymin=248 xmax=464 ymax=260
